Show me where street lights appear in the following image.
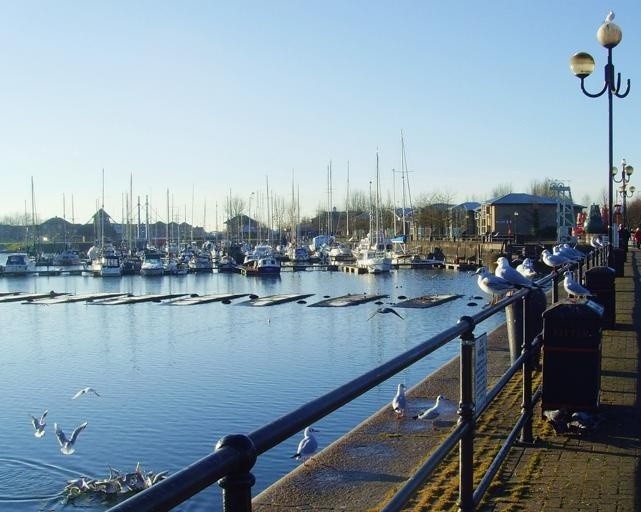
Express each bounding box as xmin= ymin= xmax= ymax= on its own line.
xmin=514 ymin=209 xmax=519 ymax=242
xmin=571 ymin=21 xmax=635 ymax=268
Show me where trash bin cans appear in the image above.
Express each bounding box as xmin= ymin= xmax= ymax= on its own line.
xmin=541 ymin=299 xmax=605 ymax=420
xmin=585 ymin=266 xmax=616 ymax=330
xmin=608 ymin=247 xmax=625 ymax=277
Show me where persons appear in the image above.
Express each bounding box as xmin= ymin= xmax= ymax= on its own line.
xmin=617 ymin=223 xmax=641 ymax=263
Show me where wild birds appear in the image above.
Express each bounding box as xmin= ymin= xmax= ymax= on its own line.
xmin=470 ymin=256 xmax=547 ymax=309
xmin=31 ymin=410 xmax=48 ymax=439
xmin=366 ymin=305 xmax=405 ymax=321
xmin=412 ymin=394 xmax=450 ymax=431
xmin=58 ymin=459 xmax=169 ymax=500
xmin=562 ymin=270 xmax=597 ymax=303
xmin=589 ymin=235 xmax=606 ymax=249
xmin=541 ymin=243 xmax=586 ymax=274
xmin=70 ymin=387 xmax=100 ymax=399
xmin=391 ymin=382 xmax=408 ymax=420
xmin=53 ymin=420 xmax=88 ymax=456
xmin=289 ymin=426 xmax=321 ymax=467
